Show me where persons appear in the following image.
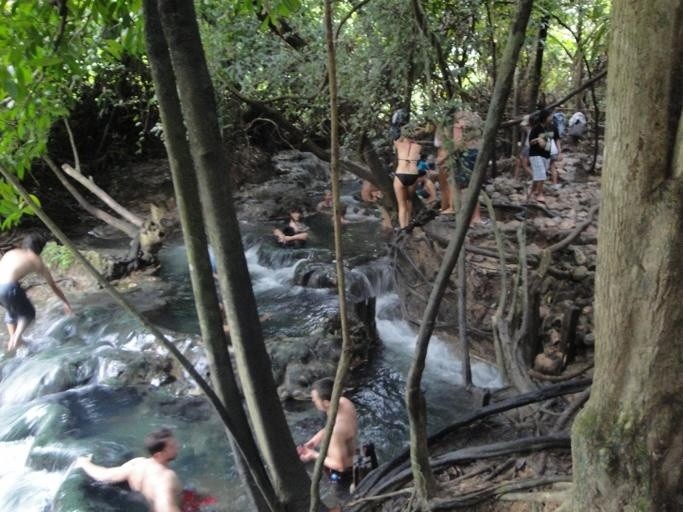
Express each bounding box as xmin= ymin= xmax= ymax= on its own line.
xmin=73 ymin=428 xmax=195 ymax=511
xmin=361 ymin=97 xmax=587 ymax=235
xmin=294 ymin=378 xmax=357 ymax=496
xmin=0 ymin=231 xmax=71 ymax=357
xmin=217 ymin=298 xmax=270 ymax=343
xmin=272 ymin=187 xmax=353 ymax=250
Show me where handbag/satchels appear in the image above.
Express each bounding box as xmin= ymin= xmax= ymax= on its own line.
xmin=545 ymin=138 xmax=559 ymax=156
